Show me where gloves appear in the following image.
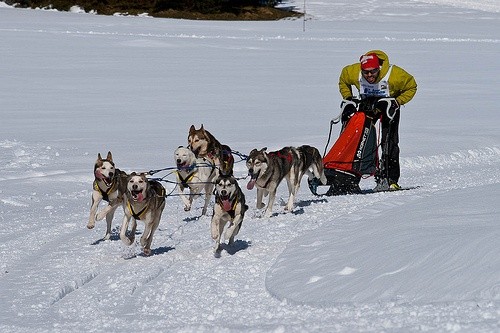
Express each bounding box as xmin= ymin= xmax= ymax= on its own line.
xmin=378 ymin=97 xmax=400 ymax=124
xmin=340 ymin=99 xmax=361 ymax=120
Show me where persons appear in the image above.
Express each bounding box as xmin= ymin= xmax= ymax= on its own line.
xmin=339 ymin=50 xmax=418 ymax=191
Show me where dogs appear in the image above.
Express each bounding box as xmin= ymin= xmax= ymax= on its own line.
xmin=175 ymin=145 xmax=216 ymax=216
xmin=296 ymin=145 xmax=327 ymax=191
xmin=188 ymin=123 xmax=235 ymax=177
xmin=246 ymin=146 xmax=305 ymax=213
xmin=210 ymin=168 xmax=249 ymax=248
xmin=87 ymin=151 xmax=132 ymax=239
xmin=119 ymin=171 xmax=167 ymax=257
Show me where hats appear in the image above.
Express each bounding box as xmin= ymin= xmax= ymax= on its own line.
xmin=360 ymin=53 xmax=380 ymax=70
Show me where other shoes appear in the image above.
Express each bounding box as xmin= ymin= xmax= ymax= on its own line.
xmin=389 ymin=183 xmax=400 ymax=191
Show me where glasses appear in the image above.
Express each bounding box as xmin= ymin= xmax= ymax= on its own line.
xmin=363 ymin=68 xmax=377 ymax=74
